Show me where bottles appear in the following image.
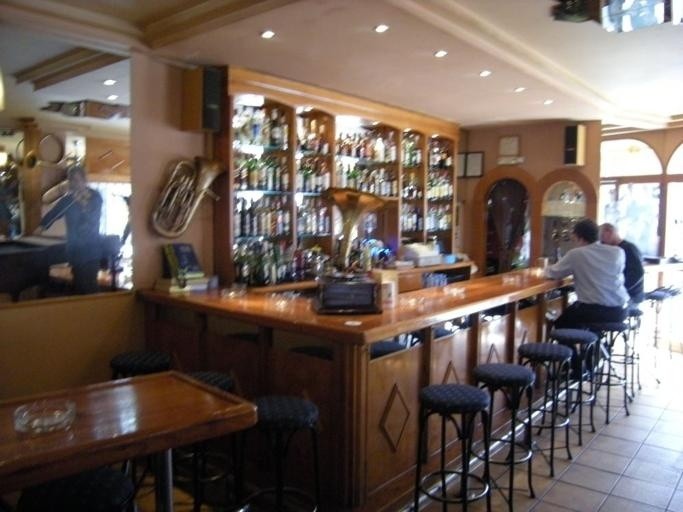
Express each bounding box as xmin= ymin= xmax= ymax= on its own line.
xmin=234 ymin=236 xmax=391 ymax=287
xmin=232 ymin=103 xmax=452 ymax=237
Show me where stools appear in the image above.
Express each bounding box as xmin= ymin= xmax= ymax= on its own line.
xmin=111 ymin=351 xmax=178 ymax=473
xmin=415 ymin=383 xmax=492 ymax=512
xmin=238 ymin=396 xmax=321 ymax=512
xmin=536 ymin=328 xmax=597 ymax=447
xmin=173 ymin=370 xmax=238 ymax=512
xmin=507 ymin=343 xmax=575 ymax=478
xmin=594 ymin=287 xmax=683 ymax=422
xmin=15 ymin=467 xmax=133 ymax=512
xmin=463 ymin=362 xmax=536 ymax=510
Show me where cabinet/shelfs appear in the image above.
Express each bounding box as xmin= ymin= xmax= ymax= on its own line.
xmin=294 ymin=82 xmax=335 ymax=278
xmin=400 ymin=120 xmax=424 ymax=253
xmin=213 ymin=72 xmax=294 ymax=282
xmin=334 ymin=104 xmax=398 ymax=263
xmin=425 ymin=127 xmax=454 ymax=260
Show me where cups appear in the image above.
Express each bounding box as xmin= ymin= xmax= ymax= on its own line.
xmin=535 ymin=256 xmax=548 ymax=277
xmin=444 ymin=255 xmax=456 ymax=265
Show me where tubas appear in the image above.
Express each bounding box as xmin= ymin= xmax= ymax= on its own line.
xmin=150 ymin=156 xmax=225 ymax=238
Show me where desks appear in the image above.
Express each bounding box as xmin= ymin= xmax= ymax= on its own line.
xmin=0 ymin=235 xmax=123 ymax=302
xmin=0 ymin=370 xmax=258 ymax=512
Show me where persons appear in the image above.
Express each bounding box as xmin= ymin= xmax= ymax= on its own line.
xmin=598 ymin=222 xmax=645 ymax=305
xmin=543 ymin=218 xmax=631 ymax=379
xmin=28 ymin=165 xmax=103 ymax=295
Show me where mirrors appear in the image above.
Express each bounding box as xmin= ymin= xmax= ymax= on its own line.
xmin=0 ymin=18 xmax=134 ymax=309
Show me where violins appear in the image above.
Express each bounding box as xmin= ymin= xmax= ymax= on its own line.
xmin=74 ymin=188 xmax=92 ymax=215
